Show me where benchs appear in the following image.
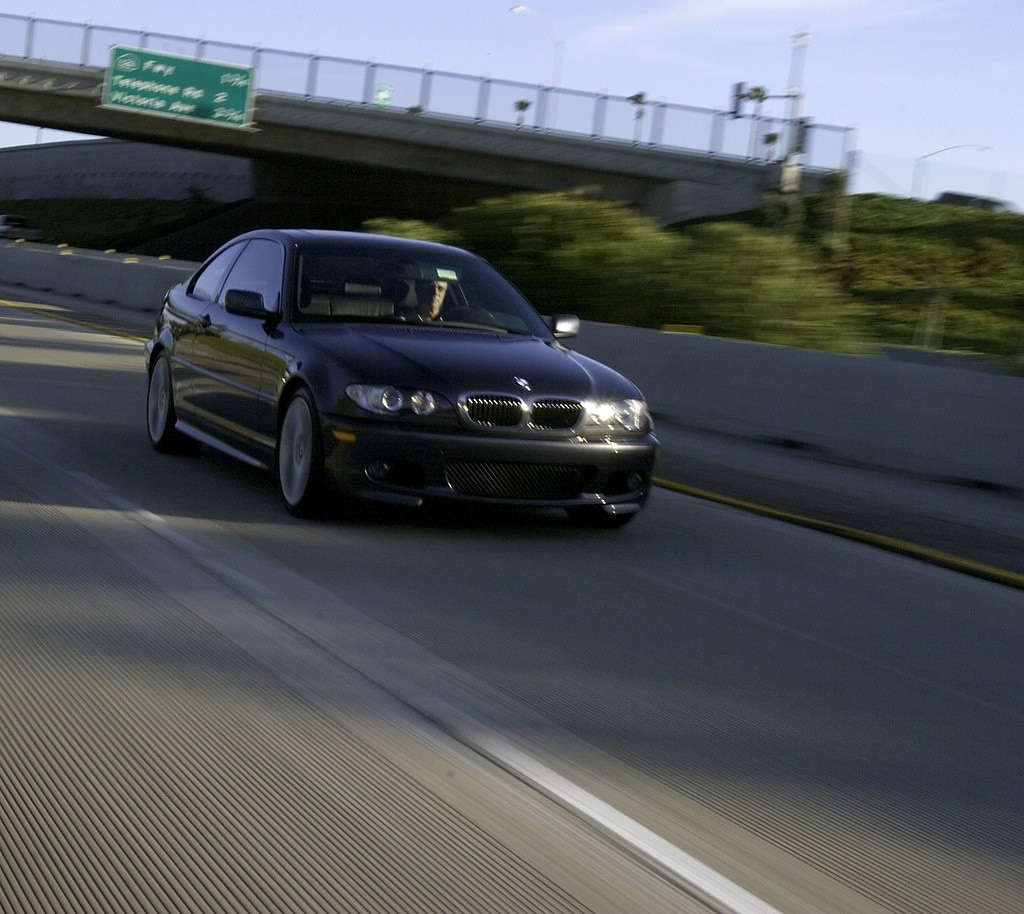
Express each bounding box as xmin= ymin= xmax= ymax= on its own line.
xmin=304 ymin=293 xmax=395 ymax=316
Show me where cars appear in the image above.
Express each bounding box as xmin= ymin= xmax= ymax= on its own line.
xmin=144 ymin=228 xmax=662 ymax=534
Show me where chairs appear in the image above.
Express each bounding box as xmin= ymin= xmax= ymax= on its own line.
xmin=382 ymin=277 xmax=413 ymax=314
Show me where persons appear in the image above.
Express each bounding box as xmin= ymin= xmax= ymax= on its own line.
xmin=410 ymin=277 xmax=448 ymax=320
